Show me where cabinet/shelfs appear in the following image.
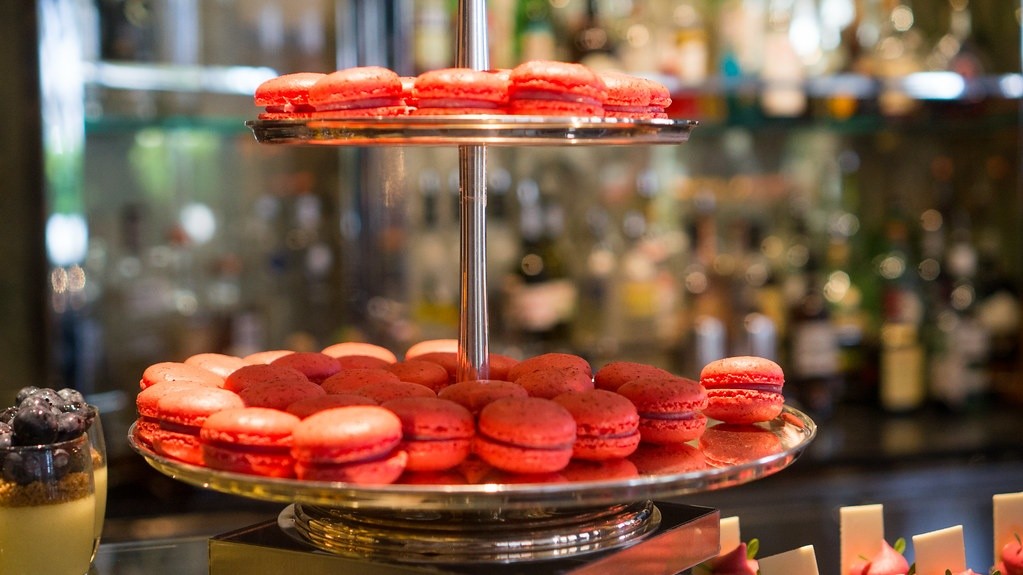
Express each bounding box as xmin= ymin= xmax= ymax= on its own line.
xmin=24 ymin=2 xmax=1022 ymax=487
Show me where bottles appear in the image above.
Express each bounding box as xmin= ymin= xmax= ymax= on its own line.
xmin=112 ymin=1 xmax=463 ymax=380
xmin=496 ymin=1 xmax=1023 ymax=423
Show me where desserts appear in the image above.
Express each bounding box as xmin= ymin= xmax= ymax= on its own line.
xmin=690 ymin=492 xmax=1023 ymax=575
xmin=137 ymin=338 xmax=784 ymax=485
xmin=251 ymin=61 xmax=674 ymax=120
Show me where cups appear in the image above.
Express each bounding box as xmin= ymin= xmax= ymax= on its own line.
xmin=0 ymin=407 xmax=107 ymax=575
